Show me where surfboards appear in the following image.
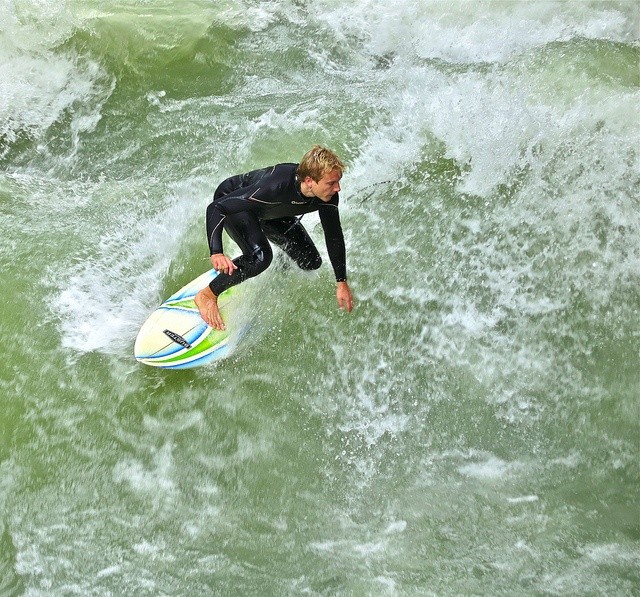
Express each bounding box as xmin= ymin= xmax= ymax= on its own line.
xmin=132 ymin=254 xmax=273 ymax=369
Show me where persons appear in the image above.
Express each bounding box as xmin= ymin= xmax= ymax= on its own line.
xmin=194 ymin=144 xmax=352 ymax=330
